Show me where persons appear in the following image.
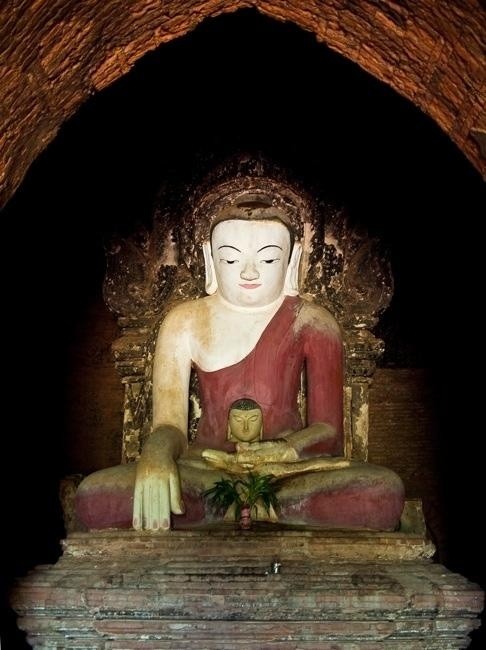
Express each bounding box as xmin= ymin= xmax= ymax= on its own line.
xmin=226 ymin=398 xmax=264 ymax=452
xmin=76 ymin=178 xmax=407 ymax=533
